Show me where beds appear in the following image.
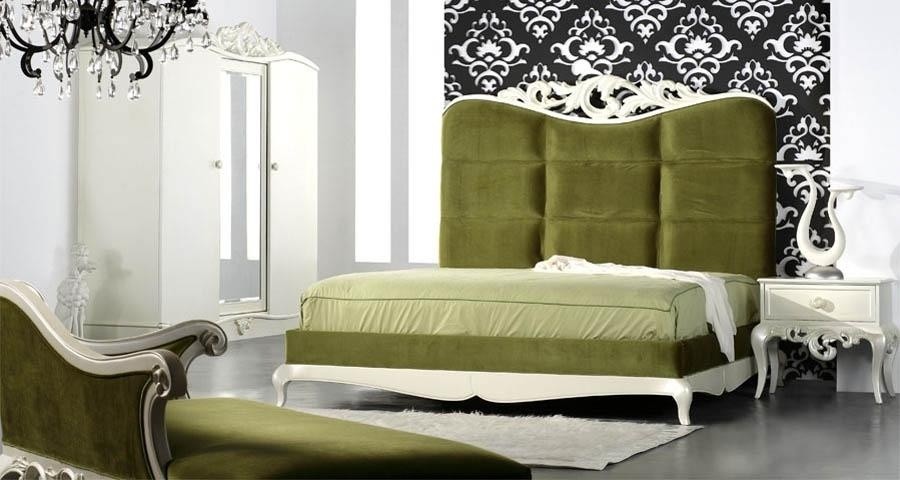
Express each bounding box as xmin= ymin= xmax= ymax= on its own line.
xmin=272 ymin=93 xmax=778 ymax=428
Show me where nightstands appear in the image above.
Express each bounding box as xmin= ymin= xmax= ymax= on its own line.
xmin=748 ymin=276 xmax=900 ymax=405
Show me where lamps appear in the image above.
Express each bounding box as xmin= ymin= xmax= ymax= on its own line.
xmin=0 ymin=0 xmax=211 ymax=99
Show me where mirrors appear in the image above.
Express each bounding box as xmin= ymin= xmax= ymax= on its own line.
xmin=216 ymin=57 xmax=267 ymax=316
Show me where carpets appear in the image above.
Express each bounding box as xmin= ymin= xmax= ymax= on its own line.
xmin=296 ymin=409 xmax=707 ymax=471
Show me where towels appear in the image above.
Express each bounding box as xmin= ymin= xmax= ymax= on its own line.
xmin=534 ymin=253 xmax=737 ymax=365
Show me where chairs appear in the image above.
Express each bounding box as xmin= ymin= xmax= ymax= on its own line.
xmin=0 ymin=280 xmax=534 ymax=480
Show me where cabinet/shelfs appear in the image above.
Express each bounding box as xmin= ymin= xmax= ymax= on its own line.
xmin=76 ymin=22 xmax=318 ymax=343
